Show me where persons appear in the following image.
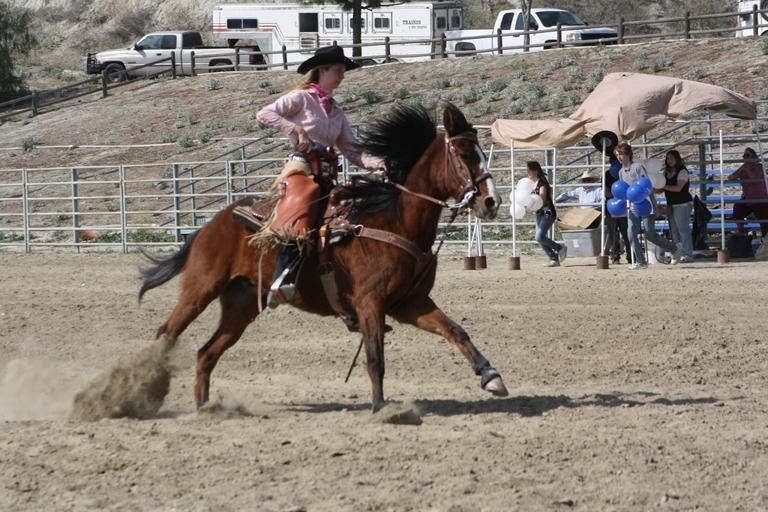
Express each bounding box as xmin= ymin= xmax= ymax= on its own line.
xmin=727 ymin=147 xmax=768 ymax=249
xmin=526 ymin=161 xmax=567 ymax=267
xmin=554 ymin=170 xmax=601 ymax=229
xmin=255 ymin=45 xmax=388 ymax=308
xmin=605 ymin=144 xmax=695 ymax=271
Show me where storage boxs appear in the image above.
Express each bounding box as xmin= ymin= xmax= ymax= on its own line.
xmin=561 ymin=228 xmax=607 ymax=257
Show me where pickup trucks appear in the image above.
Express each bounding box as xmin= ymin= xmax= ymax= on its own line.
xmin=441 ymin=7 xmax=618 ymax=61
xmin=86 ymin=30 xmax=266 ymax=85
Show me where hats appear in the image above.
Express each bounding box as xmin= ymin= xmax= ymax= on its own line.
xmin=574 ymin=171 xmax=599 ymax=183
xmin=297 ymin=45 xmax=359 ymax=74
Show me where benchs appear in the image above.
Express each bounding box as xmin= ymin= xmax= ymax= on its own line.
xmin=639 ymin=169 xmax=760 ymax=233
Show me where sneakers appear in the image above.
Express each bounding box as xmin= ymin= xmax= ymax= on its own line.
xmin=542 ymin=260 xmax=560 ymax=267
xmin=557 ymin=246 xmax=567 ymax=262
xmin=670 ymin=242 xmax=682 ymax=265
xmin=680 ymin=255 xmax=694 ymax=262
xmin=628 ymin=262 xmax=647 ymax=270
xmin=266 ymin=284 xmax=295 ymax=309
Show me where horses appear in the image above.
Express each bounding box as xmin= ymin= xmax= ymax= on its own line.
xmin=137 ymin=98 xmax=508 ymax=415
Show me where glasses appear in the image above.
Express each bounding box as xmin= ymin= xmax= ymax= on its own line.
xmin=582 ymin=178 xmax=590 ymax=182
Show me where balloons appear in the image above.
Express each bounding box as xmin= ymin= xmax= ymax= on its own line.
xmin=509 ymin=177 xmax=543 ymax=220
